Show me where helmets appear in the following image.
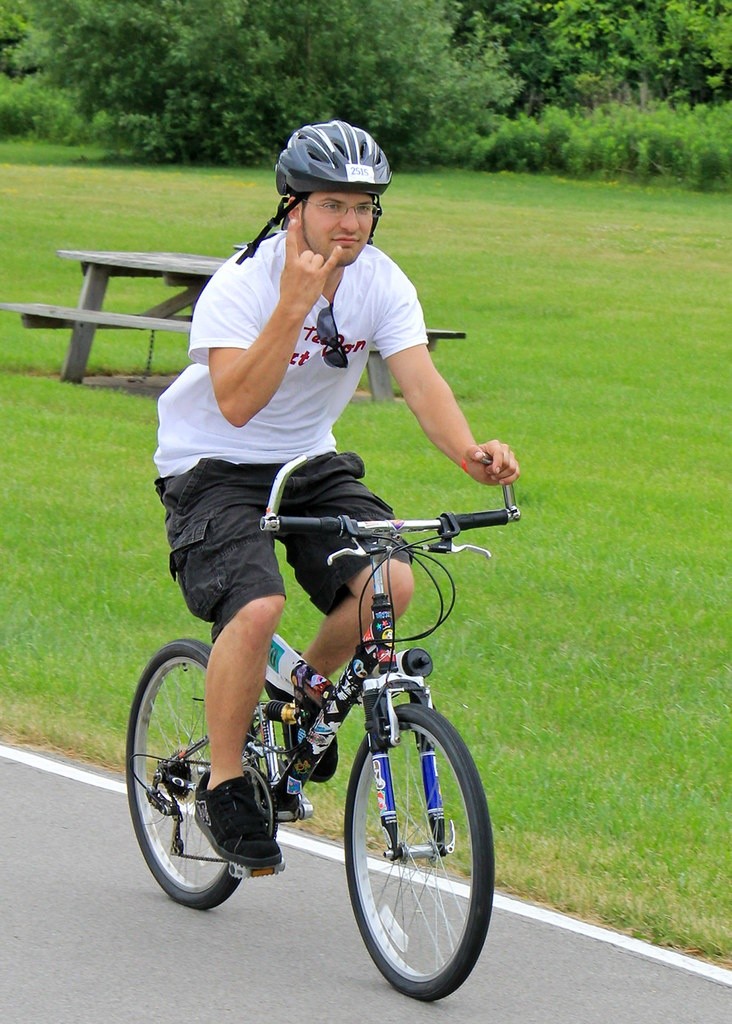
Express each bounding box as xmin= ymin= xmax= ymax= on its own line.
xmin=276 ymin=120 xmax=391 ymax=197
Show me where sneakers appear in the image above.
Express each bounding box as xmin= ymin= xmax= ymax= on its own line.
xmin=194 ymin=769 xmax=282 ymax=868
xmin=265 ymin=649 xmax=339 ymax=783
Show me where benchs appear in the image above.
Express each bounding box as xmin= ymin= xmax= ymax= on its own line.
xmin=0 ymin=302 xmax=192 ymax=385
xmin=425 ymin=330 xmax=468 ymax=349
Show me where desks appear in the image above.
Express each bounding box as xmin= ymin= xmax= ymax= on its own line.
xmin=55 ymin=249 xmax=397 ymax=403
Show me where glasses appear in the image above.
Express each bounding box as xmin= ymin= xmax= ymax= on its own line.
xmin=301 ymin=198 xmax=378 ymax=217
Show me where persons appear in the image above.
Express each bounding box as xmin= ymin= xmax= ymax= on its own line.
xmin=154 ymin=118 xmax=521 ymax=869
